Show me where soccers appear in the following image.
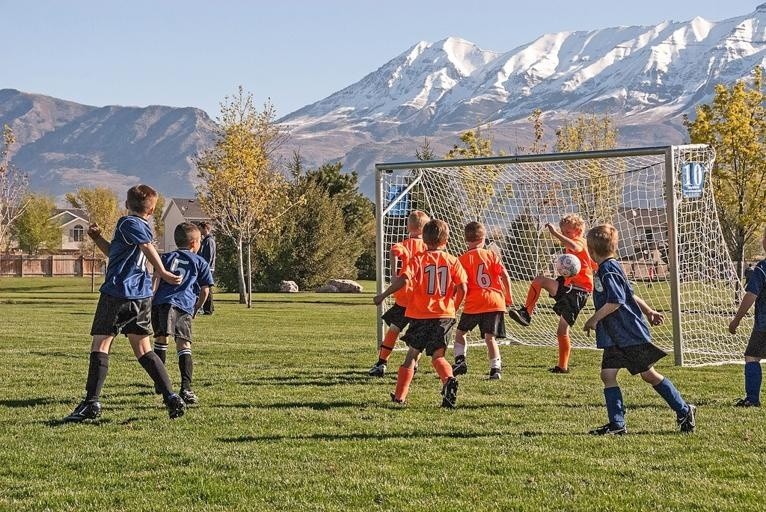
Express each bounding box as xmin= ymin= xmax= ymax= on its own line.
xmin=556 ymin=254 xmax=582 ymax=277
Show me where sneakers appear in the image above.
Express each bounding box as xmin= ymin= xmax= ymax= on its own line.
xmin=734 ymin=397 xmax=761 ymax=407
xmin=547 ymin=366 xmax=569 ymax=373
xmin=451 ymin=355 xmax=467 ymax=376
xmin=588 ymin=422 xmax=627 ymax=436
xmin=509 ymin=304 xmax=531 ymax=326
xmin=153 ymin=383 xmax=199 ymax=419
xmin=677 ymin=405 xmax=696 ymax=433
xmin=489 ymin=367 xmax=502 ymax=380
xmin=50 ymin=400 xmax=102 ymax=428
xmin=368 ymin=365 xmax=386 ymax=377
xmin=440 ymin=376 xmax=458 ymax=409
xmin=198 ymin=308 xmax=214 ymax=316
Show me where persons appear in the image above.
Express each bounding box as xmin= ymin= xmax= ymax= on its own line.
xmin=365 ymin=210 xmax=431 ymax=377
xmin=581 ymin=223 xmax=697 ymax=436
xmin=197 ymin=222 xmax=216 ymax=316
xmin=373 ymin=219 xmax=469 ymax=410
xmin=150 ymin=221 xmax=217 ymax=406
xmin=743 ymin=262 xmax=754 ymax=288
xmin=647 ymin=264 xmax=656 ymax=288
xmin=727 ymin=231 xmax=765 ymax=408
xmin=508 ymin=212 xmax=599 ymax=373
xmin=62 ymin=183 xmax=187 ymax=422
xmin=451 ymin=221 xmax=513 ymax=378
